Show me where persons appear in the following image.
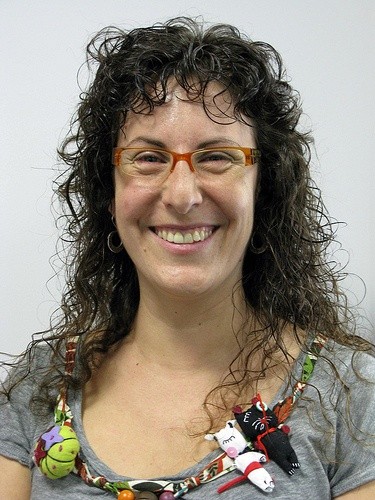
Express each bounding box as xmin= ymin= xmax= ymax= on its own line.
xmin=0 ymin=15 xmax=374 ymax=500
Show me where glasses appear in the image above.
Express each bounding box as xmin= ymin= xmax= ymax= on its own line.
xmin=109 ymin=145 xmax=263 ymax=179
